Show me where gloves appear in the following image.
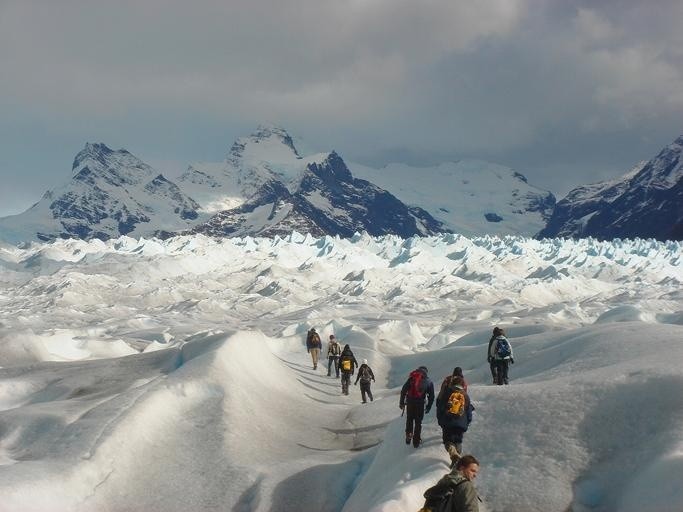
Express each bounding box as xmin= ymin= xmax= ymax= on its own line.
xmin=511 ymin=359 xmax=514 ymax=364
xmin=425 ymin=405 xmax=431 ymax=414
xmin=488 ymin=357 xmax=491 ymax=362
xmin=399 ymin=403 xmax=405 ymax=409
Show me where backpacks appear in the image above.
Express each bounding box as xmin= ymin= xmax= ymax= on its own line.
xmin=342 ymin=360 xmax=351 ymax=370
xmin=308 ymin=333 xmax=320 ymax=346
xmin=424 ymin=478 xmax=469 ymax=511
xmin=361 ymin=368 xmax=372 ymax=384
xmin=441 ymin=393 xmax=466 ymax=418
xmin=408 ymin=370 xmax=427 ymax=399
xmin=495 ymin=339 xmax=511 ymax=357
xmin=331 ymin=343 xmax=342 ymax=356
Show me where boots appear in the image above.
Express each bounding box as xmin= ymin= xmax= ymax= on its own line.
xmin=493 ymin=375 xmax=509 ymax=385
xmin=413 ymin=433 xmax=422 ymax=448
xmin=406 ymin=432 xmax=413 ymax=445
xmin=444 ymin=442 xmax=461 ymax=470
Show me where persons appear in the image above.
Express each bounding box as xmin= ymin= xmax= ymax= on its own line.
xmin=326 ymin=334 xmax=341 ymax=377
xmin=354 ymin=358 xmax=376 ymax=404
xmin=304 ymin=327 xmax=322 ymax=371
xmin=436 ymin=366 xmax=466 ymax=407
xmin=422 ymin=455 xmax=481 ymax=512
xmin=398 ymin=366 xmax=434 ymax=447
xmin=337 ymin=344 xmax=358 ymax=396
xmin=436 ymin=377 xmax=471 ymax=465
xmin=487 ymin=327 xmax=502 ymax=383
xmin=490 ymin=329 xmax=514 ymax=385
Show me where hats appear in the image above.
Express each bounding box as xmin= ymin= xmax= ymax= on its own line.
xmin=362 ymin=359 xmax=368 ymax=364
